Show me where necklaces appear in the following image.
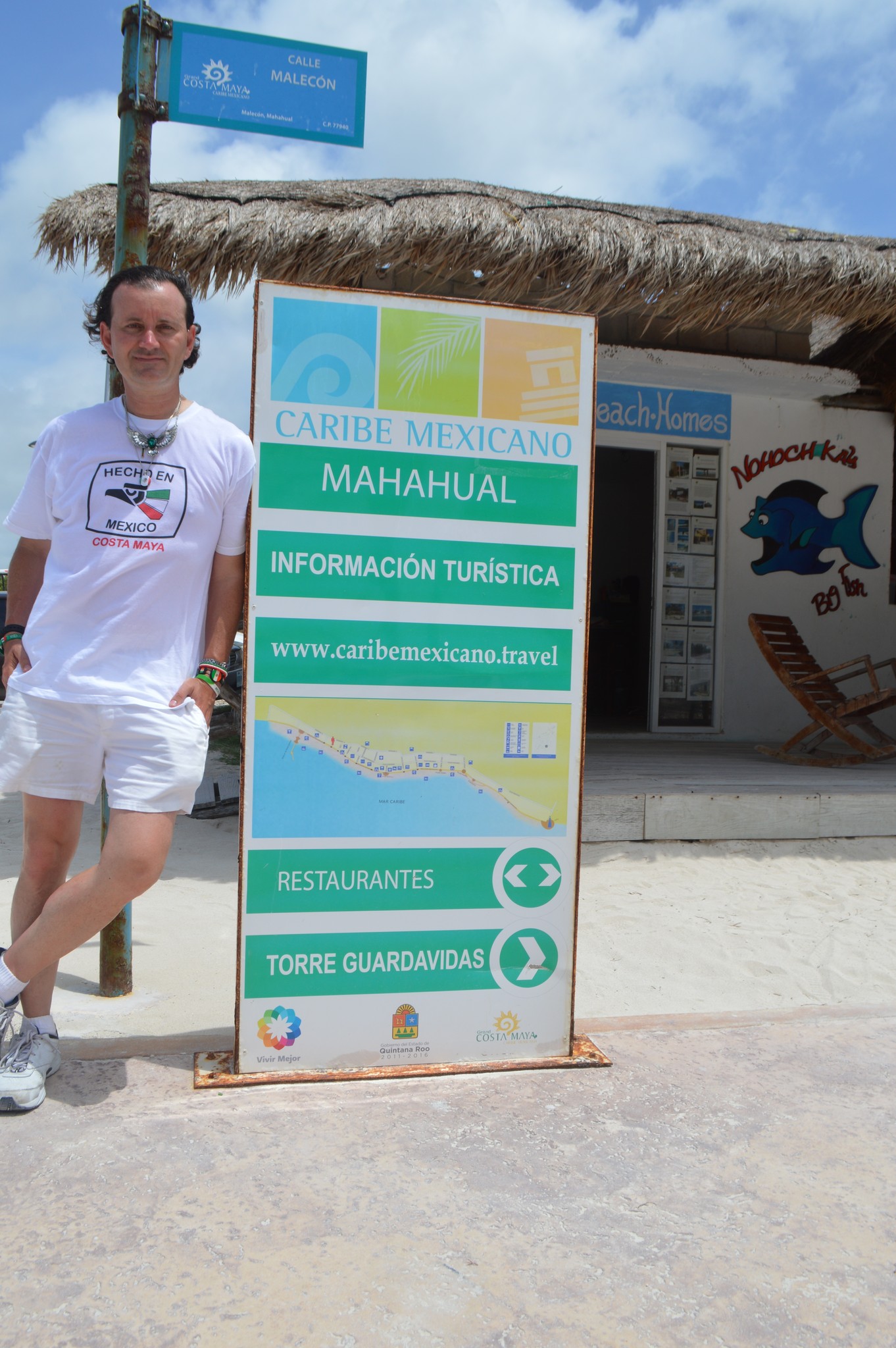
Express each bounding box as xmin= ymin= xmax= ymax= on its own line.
xmin=123 ymin=393 xmax=182 ymax=486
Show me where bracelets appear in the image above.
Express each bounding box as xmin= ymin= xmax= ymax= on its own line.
xmin=0 ymin=624 xmax=26 ymax=656
xmin=196 ymin=662 xmax=228 ymax=697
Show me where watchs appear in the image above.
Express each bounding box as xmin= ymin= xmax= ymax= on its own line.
xmin=202 ymin=659 xmax=228 ymax=670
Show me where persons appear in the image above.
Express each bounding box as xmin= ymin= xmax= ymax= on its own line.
xmin=0 ymin=267 xmax=254 ymax=1116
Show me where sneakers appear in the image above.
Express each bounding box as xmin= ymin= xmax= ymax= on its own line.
xmin=0 ymin=946 xmax=36 ymax=1046
xmin=0 ymin=1025 xmax=61 ymax=1112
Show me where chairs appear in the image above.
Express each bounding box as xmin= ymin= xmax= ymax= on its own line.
xmin=749 ymin=613 xmax=896 ymax=768
